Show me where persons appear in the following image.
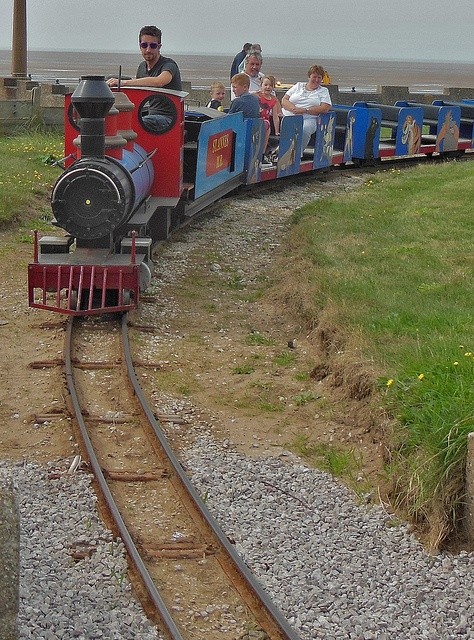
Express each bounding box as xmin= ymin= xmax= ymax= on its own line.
xmin=252 ymin=76 xmax=280 ymax=167
xmin=230 ymin=43 xmax=253 ymax=80
xmin=105 ymin=26 xmax=182 ymax=91
xmin=238 ymin=44 xmax=262 ymax=73
xmin=281 ymin=65 xmax=332 ymax=163
xmin=231 ymin=50 xmax=275 ymax=115
xmin=228 ymin=73 xmax=260 ymax=122
xmin=205 ymin=82 xmax=226 ymax=112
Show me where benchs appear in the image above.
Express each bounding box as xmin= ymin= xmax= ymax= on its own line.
xmin=355 ymin=100 xmax=423 ymax=155
xmin=316 ymin=104 xmax=382 ymax=162
xmin=435 ymin=99 xmax=473 ymax=146
xmin=396 ymin=101 xmax=460 ymax=153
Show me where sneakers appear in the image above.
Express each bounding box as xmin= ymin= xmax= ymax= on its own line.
xmin=262 ymin=153 xmax=272 ymax=166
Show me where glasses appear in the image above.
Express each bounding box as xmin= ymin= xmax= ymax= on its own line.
xmin=139 ymin=42 xmax=161 ymax=49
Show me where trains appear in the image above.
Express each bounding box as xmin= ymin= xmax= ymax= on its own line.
xmin=23 ymin=62 xmax=474 ymax=317
